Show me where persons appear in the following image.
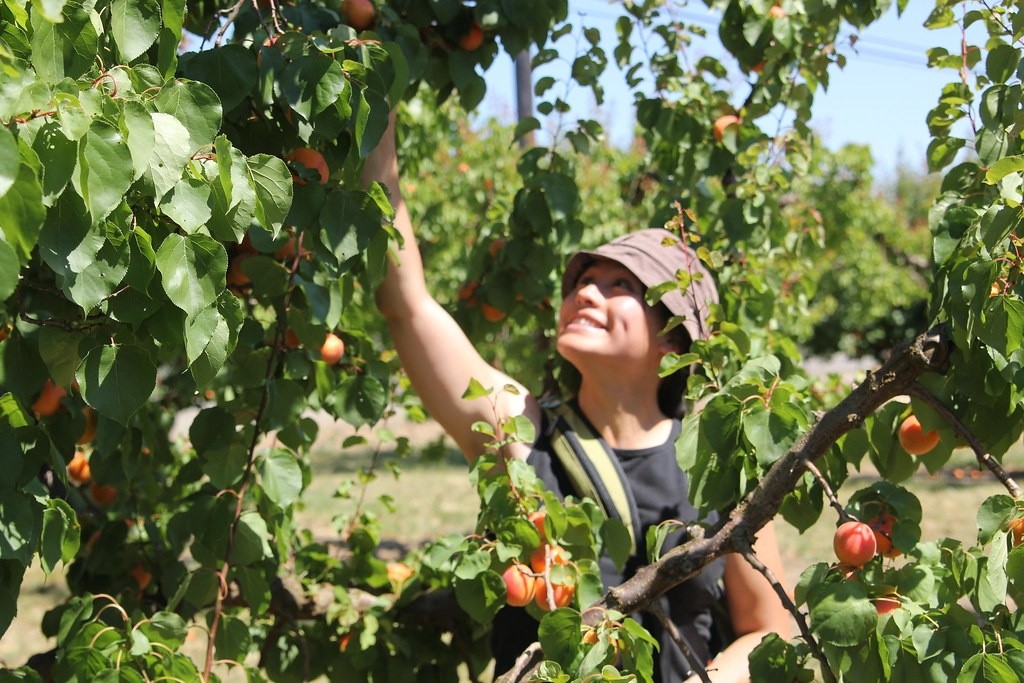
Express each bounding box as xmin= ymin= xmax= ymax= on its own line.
xmin=352 ymin=92 xmax=794 ymax=683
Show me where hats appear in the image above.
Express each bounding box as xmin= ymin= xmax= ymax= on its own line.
xmin=562 ymin=227 xmax=719 ymax=393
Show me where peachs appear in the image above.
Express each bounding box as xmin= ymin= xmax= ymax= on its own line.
xmin=28 ymin=0 xmax=1023 ymax=667
